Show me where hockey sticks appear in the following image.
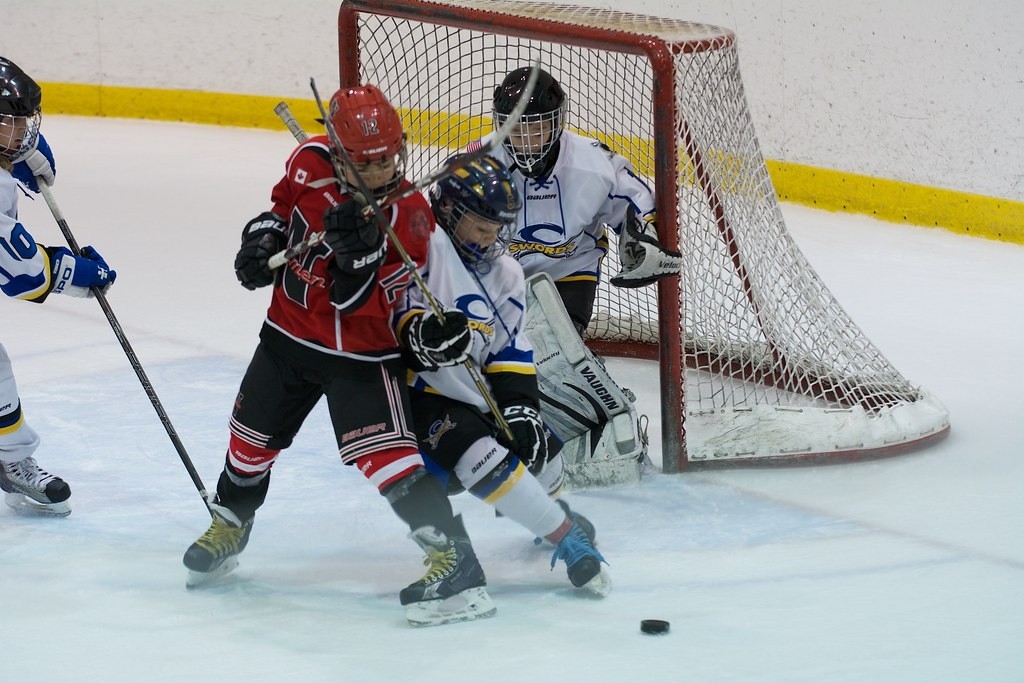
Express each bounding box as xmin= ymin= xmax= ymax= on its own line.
xmin=301 ymin=76 xmax=521 ymax=453
xmin=274 ymin=101 xmax=310 ymax=144
xmin=265 ymin=55 xmax=542 ymax=270
xmin=27 ymin=150 xmax=244 ymax=590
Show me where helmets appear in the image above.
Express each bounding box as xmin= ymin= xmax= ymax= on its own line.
xmin=428 ymin=153 xmax=524 ymax=270
xmin=0 ymin=55 xmax=41 ymax=159
xmin=491 ymin=67 xmax=568 ymax=176
xmin=322 ymin=84 xmax=407 ymax=204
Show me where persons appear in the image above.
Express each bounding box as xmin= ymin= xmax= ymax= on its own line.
xmin=0 ymin=55 xmax=117 ymax=517
xmin=388 ymin=150 xmax=618 ymax=599
xmin=452 ymin=63 xmax=663 ymax=338
xmin=181 ymin=82 xmax=501 ymax=627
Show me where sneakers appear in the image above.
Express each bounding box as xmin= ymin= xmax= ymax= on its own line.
xmin=556 ymin=497 xmax=604 ymax=588
xmin=0 ymin=460 xmax=72 ymax=518
xmin=400 ymin=537 xmax=497 ymax=626
xmin=183 ymin=510 xmax=255 ymax=588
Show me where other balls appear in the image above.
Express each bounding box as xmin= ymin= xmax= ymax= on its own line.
xmin=639 ymin=619 xmax=670 ymax=634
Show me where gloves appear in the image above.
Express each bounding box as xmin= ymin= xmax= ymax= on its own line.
xmin=496 ymin=404 xmax=548 ymax=477
xmin=234 ymin=213 xmax=297 ymax=291
xmin=406 ymin=309 xmax=473 ymax=371
xmin=10 ymin=133 xmax=56 ymax=192
xmin=323 ymin=202 xmax=386 ymax=276
xmin=45 ymin=246 xmax=116 ymax=299
